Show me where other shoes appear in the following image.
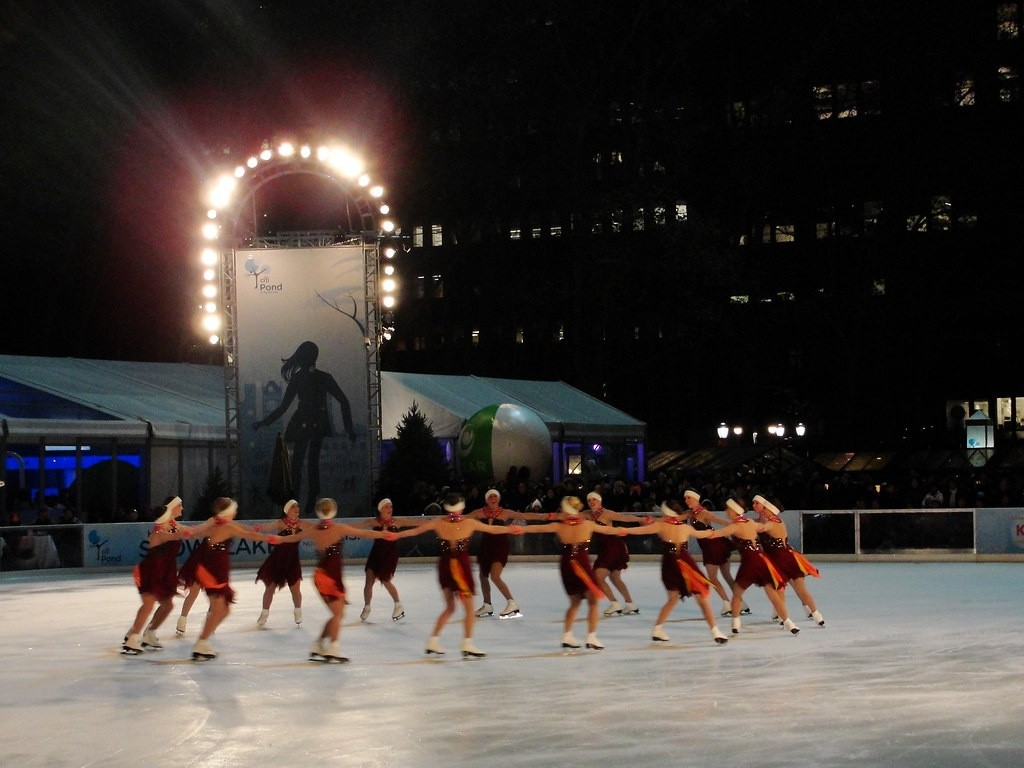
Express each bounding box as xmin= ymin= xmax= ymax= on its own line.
xmin=652 ymin=625 xmax=670 ymax=641
xmin=392 ymin=602 xmax=404 ymax=621
xmin=779 ymin=617 xmax=800 ymax=637
xmin=802 ymin=604 xmax=826 ymax=629
xmin=473 ymin=602 xmax=493 ymax=617
xmin=294 ymin=608 xmax=301 ymax=628
xmin=176 ymin=616 xmax=186 ymax=635
xmin=772 ymin=607 xmax=779 ymax=622
xmin=721 ymin=600 xmax=732 ymax=616
xmin=256 ymin=609 xmax=269 ymax=630
xmin=740 ymin=601 xmax=752 ymax=615
xmin=309 ymin=639 xmax=349 ymax=664
xmin=424 ymin=636 xmax=445 ymax=654
xmin=120 ymin=634 xmax=144 ymax=655
xmin=731 ymin=617 xmax=741 ymax=633
xmin=141 ymin=629 xmax=162 ymax=648
xmin=563 ymin=631 xmax=582 ymax=648
xmin=191 ymin=639 xmax=216 ymax=660
xmin=361 ymin=606 xmax=371 ymax=622
xmin=711 ymin=626 xmax=728 ymax=643
xmin=500 ymin=598 xmax=519 ymax=616
xmin=462 ymin=638 xmax=484 ymax=657
xmin=604 ymin=600 xmax=622 ymax=616
xmin=586 ymin=631 xmax=605 ymax=650
xmin=621 ymin=602 xmax=640 ymax=615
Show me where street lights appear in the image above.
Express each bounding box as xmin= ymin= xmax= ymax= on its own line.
xmin=714 ymin=419 xmax=807 ymax=469
xmin=773 ymin=422 xmax=788 ymax=477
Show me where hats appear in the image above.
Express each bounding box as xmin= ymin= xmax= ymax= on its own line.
xmin=644 ymin=481 xmax=652 ymax=485
xmin=603 ymin=484 xmax=610 ymax=488
xmin=614 ymin=481 xmax=625 ymax=488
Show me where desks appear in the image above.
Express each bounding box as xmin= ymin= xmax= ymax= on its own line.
xmin=14 ymin=536 xmax=60 ymax=570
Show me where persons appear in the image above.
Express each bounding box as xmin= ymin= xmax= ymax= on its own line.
xmin=32 ymin=508 xmax=56 ymax=524
xmin=60 ymin=510 xmax=78 ymax=524
xmin=400 ymin=460 xmax=1024 ymax=554
xmin=120 ymin=487 xmax=825 ymax=662
xmin=9 ymin=512 xmax=22 ymax=526
